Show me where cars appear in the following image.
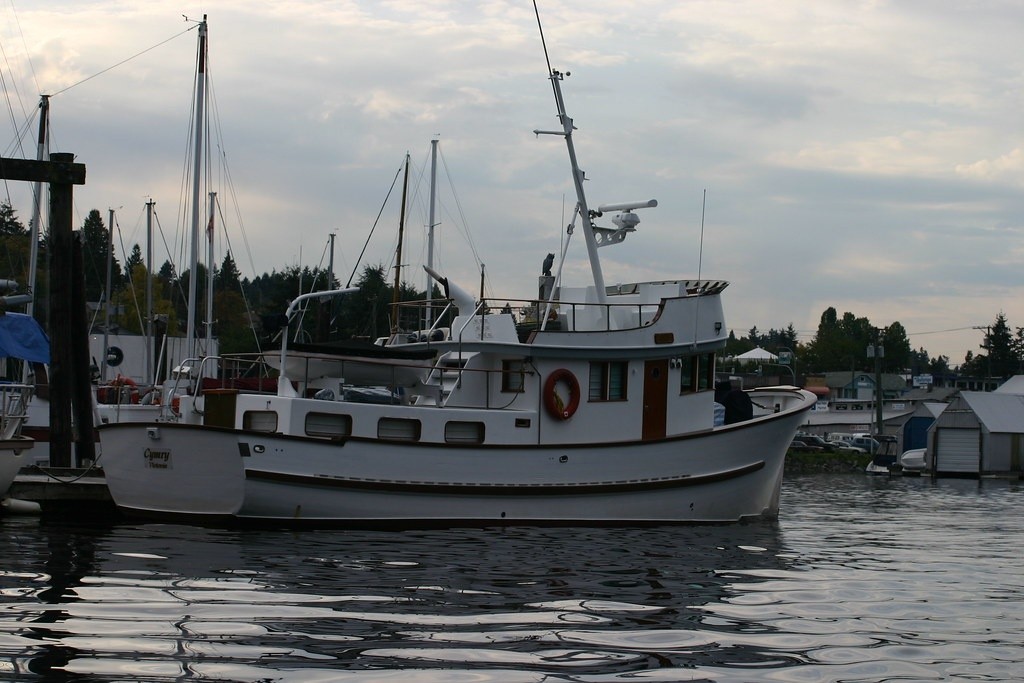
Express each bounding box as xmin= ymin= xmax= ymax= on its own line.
xmin=851 ymin=437 xmax=880 ymax=452
xmin=831 ymin=440 xmax=868 ymax=456
xmin=790 ymin=441 xmax=825 ymax=455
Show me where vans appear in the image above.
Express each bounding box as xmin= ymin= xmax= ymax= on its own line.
xmin=827 ymin=432 xmax=897 ymax=441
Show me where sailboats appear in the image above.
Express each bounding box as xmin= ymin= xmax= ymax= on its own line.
xmin=1 ymin=0 xmax=819 ymax=530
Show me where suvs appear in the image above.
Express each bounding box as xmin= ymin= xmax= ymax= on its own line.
xmin=795 ymin=435 xmax=837 ymax=452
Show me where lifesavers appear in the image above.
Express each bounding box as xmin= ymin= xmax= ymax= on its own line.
xmin=101 ymin=378 xmax=139 ymax=405
xmin=544 ymin=368 xmax=580 ymax=419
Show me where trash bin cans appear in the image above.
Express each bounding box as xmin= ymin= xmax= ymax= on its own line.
xmin=201 ymin=389 xmax=240 ymax=429
